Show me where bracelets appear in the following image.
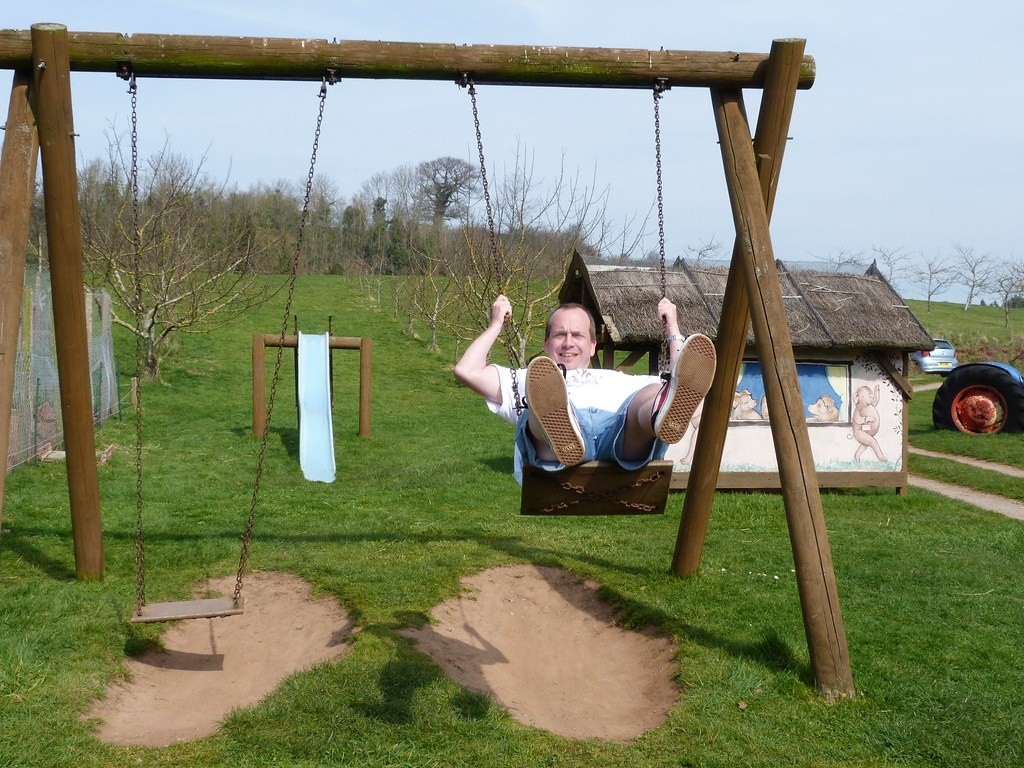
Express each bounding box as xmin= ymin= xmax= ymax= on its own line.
xmin=668 ymin=335 xmax=685 ymax=345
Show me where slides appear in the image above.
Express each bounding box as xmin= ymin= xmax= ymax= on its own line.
xmin=297 ymin=330 xmax=336 ymax=483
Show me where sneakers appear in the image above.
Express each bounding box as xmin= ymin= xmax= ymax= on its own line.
xmin=525 ymin=355 xmax=586 ymax=468
xmin=650 ymin=333 xmax=717 ymax=444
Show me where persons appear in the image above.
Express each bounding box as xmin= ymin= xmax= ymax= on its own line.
xmin=454 ymin=294 xmax=716 ymax=488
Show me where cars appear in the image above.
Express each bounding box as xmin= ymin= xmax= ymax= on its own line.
xmin=910 ymin=339 xmax=958 ymax=372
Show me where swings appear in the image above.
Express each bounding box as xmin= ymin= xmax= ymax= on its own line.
xmin=123 ymin=75 xmax=326 ymax=623
xmin=467 ymin=81 xmax=675 ymax=516
